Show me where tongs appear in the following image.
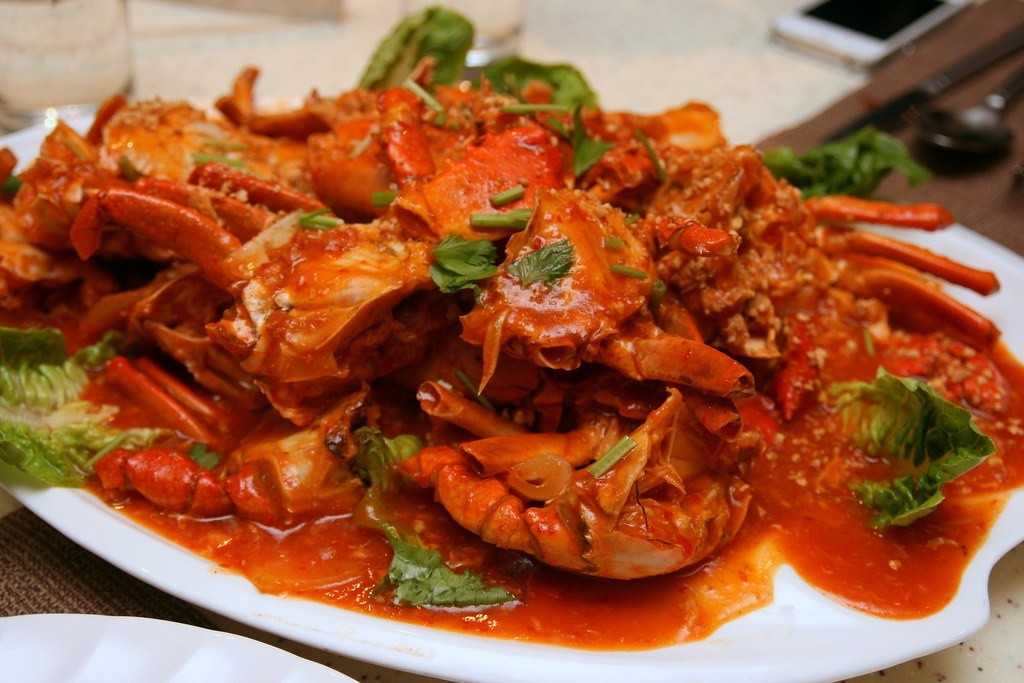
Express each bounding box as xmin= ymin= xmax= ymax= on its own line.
xmin=750 ymin=1 xmax=1024 ymax=198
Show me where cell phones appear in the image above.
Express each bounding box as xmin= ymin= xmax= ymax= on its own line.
xmin=772 ymin=0 xmax=973 ymax=67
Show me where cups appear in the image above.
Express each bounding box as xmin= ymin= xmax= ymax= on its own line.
xmin=400 ymin=0 xmax=524 ymax=67
xmin=0 ymin=0 xmax=135 ymax=131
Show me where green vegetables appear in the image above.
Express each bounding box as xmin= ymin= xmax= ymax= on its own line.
xmin=0 ymin=7 xmax=1000 ymax=611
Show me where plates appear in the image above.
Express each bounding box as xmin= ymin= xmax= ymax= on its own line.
xmin=0 ymin=613 xmax=361 ymax=683
xmin=0 ymin=103 xmax=1024 ymax=683
